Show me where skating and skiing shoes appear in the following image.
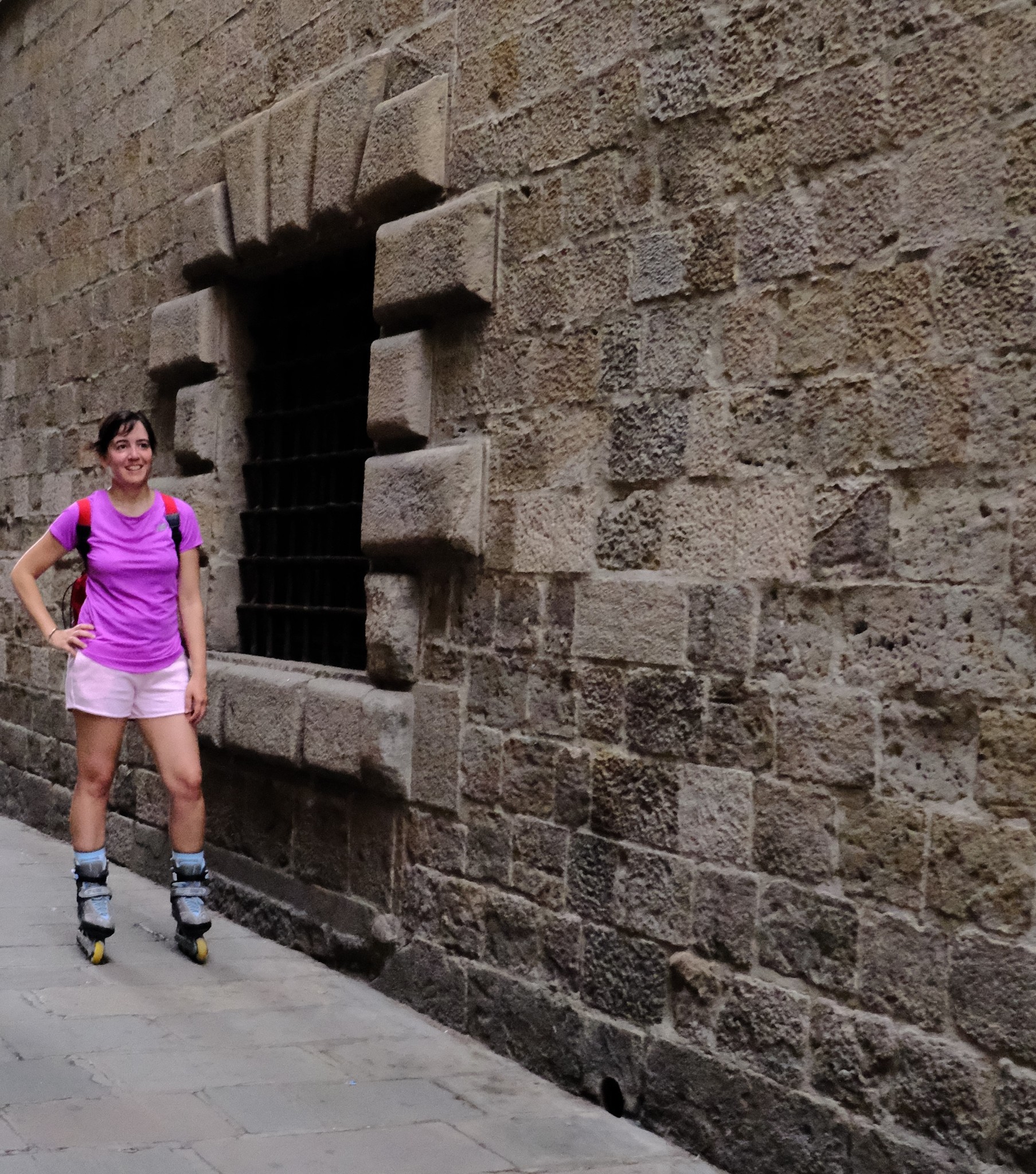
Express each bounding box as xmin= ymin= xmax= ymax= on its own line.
xmin=169 ymin=857 xmax=211 ymax=964
xmin=71 ymin=857 xmax=115 ymax=965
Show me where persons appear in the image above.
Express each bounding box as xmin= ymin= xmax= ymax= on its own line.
xmin=10 ymin=411 xmax=213 ymax=966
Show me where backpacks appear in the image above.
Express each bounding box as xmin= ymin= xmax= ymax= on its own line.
xmin=61 ymin=493 xmax=183 ymax=630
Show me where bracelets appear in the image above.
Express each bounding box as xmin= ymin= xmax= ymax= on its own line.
xmin=46 ymin=628 xmax=60 ymax=647
